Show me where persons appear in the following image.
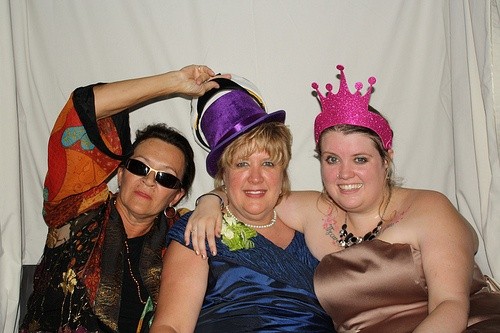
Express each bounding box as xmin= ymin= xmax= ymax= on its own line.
xmin=184 ymin=65 xmax=500 ymax=333
xmin=151 ymin=75 xmax=336 ymax=333
xmin=19 ymin=64 xmax=232 ymax=333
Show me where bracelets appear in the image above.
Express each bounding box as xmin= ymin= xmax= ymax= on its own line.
xmin=195 ymin=193 xmax=224 ymax=210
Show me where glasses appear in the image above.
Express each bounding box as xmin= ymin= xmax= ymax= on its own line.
xmin=125 ymin=158 xmax=183 ymax=190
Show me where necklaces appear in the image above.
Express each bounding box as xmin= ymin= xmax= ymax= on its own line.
xmin=225 ymin=204 xmax=277 ymax=228
xmin=126 ymin=241 xmax=147 ymax=304
xmin=339 ymin=211 xmax=386 ymax=248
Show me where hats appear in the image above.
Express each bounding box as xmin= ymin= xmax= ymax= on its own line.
xmin=189 ymin=72 xmax=268 ymax=153
xmin=200 ymin=90 xmax=287 ymax=179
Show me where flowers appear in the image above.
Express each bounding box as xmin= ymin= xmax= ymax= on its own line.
xmin=220 ymin=216 xmax=257 ymax=251
